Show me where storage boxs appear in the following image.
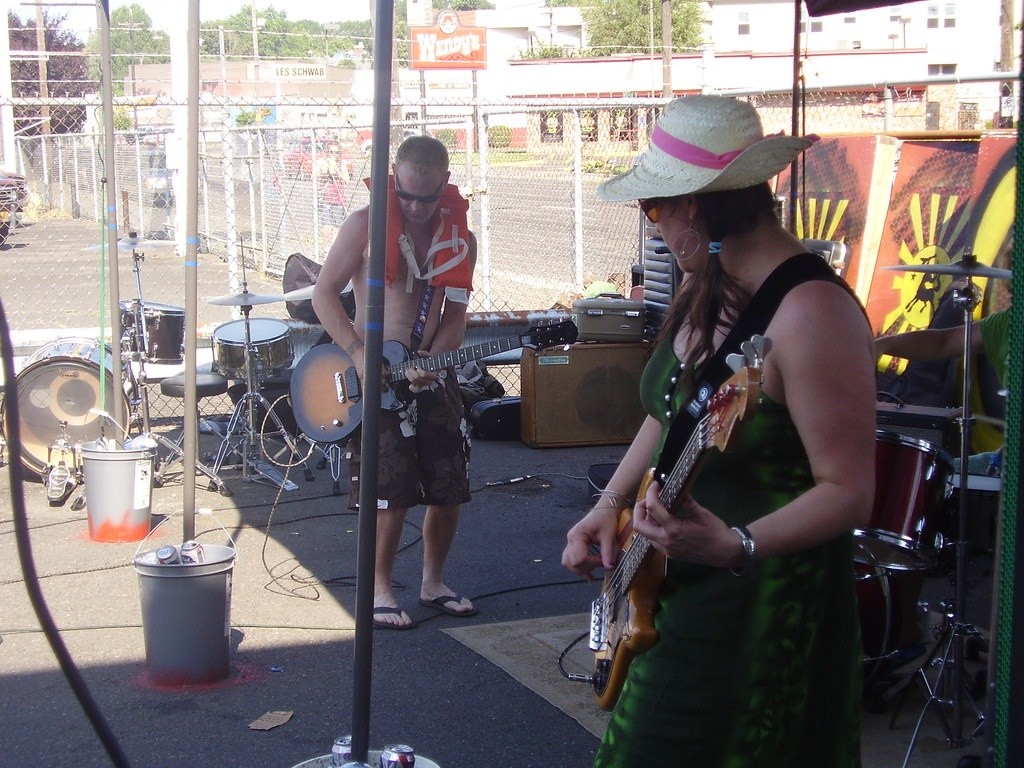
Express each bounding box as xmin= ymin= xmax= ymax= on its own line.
xmin=571 ymin=293 xmax=646 ymax=341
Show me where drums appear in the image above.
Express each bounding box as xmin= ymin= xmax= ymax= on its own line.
xmin=120 ymin=299 xmax=184 ymax=366
xmin=0 ymin=337 xmax=140 ymax=478
xmin=852 ymin=563 xmax=903 ymax=701
xmin=846 ymin=430 xmax=958 ymax=572
xmin=210 ymin=317 xmax=294 ymax=377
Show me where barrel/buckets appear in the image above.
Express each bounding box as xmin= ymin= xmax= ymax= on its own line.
xmin=293 ymin=751 xmax=440 ymax=768
xmin=81 ymin=441 xmax=157 ymax=544
xmin=134 ymin=545 xmax=236 ymax=684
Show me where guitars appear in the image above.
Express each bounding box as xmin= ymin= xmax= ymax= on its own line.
xmin=589 ymin=332 xmax=774 ymax=713
xmin=289 ymin=316 xmax=580 ymax=445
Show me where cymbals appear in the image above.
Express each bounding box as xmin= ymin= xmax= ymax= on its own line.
xmin=880 ymin=260 xmax=1015 ymax=281
xmin=204 ymin=294 xmax=282 ymax=307
xmin=283 ymin=283 xmax=352 ymax=302
xmin=80 ymin=237 xmax=184 ymax=250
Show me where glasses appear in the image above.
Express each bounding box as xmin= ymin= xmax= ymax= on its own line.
xmin=395 ymin=173 xmax=443 ymax=203
xmin=638 ymin=198 xmax=663 ymax=223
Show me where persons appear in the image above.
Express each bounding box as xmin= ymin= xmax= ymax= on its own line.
xmin=313 ymin=141 xmax=351 ymax=254
xmin=311 ymin=135 xmax=478 ymax=630
xmin=561 ymin=94 xmax=879 ymax=768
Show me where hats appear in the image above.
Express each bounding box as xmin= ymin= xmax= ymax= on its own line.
xmin=596 ymin=94 xmax=814 ymax=205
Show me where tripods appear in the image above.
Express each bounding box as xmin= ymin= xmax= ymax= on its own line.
xmin=890 ymin=277 xmax=992 ymax=768
xmin=208 ymin=306 xmax=316 ymax=493
xmin=71 ymin=243 xmax=233 ymax=514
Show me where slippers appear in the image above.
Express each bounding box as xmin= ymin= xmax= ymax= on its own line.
xmin=419 ymin=592 xmax=477 ymax=618
xmin=373 ymin=607 xmax=414 ymax=630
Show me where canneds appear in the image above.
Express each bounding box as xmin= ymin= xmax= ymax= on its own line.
xmin=156 ymin=545 xmax=181 ymax=564
xmin=331 ymin=734 xmax=353 ymax=768
xmin=179 ymin=541 xmax=206 ymax=565
xmin=379 ymin=744 xmax=415 ymax=768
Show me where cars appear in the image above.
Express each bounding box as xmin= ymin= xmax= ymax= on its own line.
xmin=0 ymin=168 xmax=29 ymax=246
xmin=286 ymin=138 xmax=354 ymax=180
xmin=62 ymin=146 xmax=205 ymax=205
xmin=359 ymin=128 xmax=438 ymax=159
xmin=125 ymin=126 xmax=176 ymax=144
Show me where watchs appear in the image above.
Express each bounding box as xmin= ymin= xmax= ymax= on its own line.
xmin=730 ymin=526 xmax=758 ymax=577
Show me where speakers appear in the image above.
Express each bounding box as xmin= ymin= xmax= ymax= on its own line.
xmin=520 ymin=343 xmax=652 ymax=450
